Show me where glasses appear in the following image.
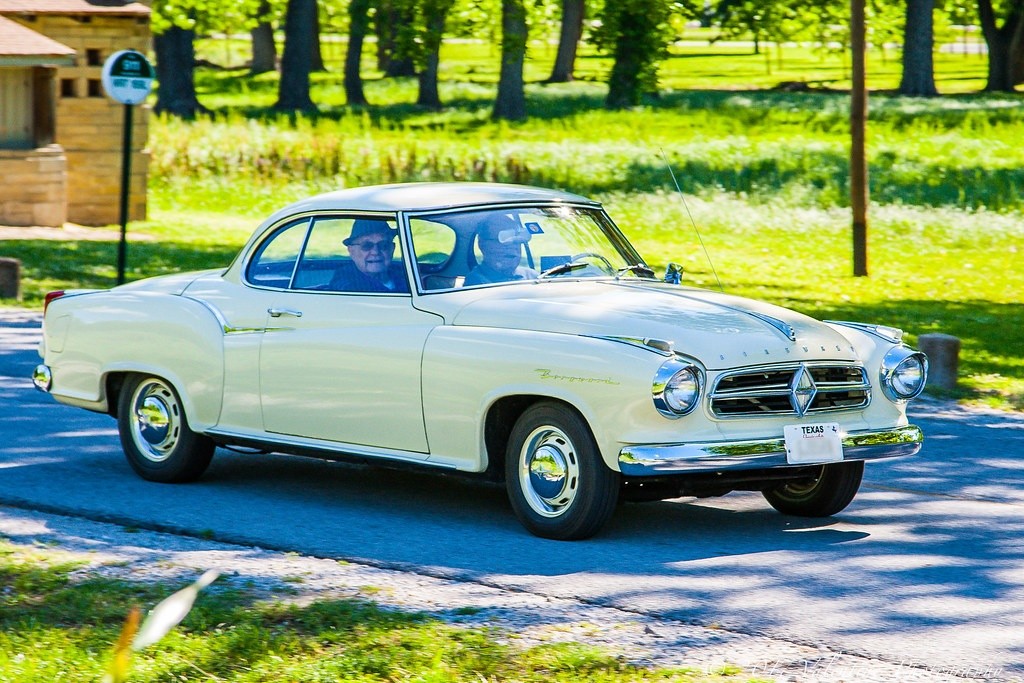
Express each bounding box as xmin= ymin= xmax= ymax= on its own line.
xmin=484 ymin=228 xmax=531 ymax=244
xmin=348 ymin=240 xmax=390 ymax=251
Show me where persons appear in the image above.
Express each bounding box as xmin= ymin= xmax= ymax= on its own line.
xmin=462 ymin=213 xmax=525 ymax=287
xmin=326 ymin=219 xmax=410 ymax=294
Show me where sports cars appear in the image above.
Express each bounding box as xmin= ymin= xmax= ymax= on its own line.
xmin=31 ymin=182 xmax=929 ymax=550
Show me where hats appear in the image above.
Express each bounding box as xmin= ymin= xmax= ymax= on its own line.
xmin=343 ymin=219 xmax=396 ymax=246
